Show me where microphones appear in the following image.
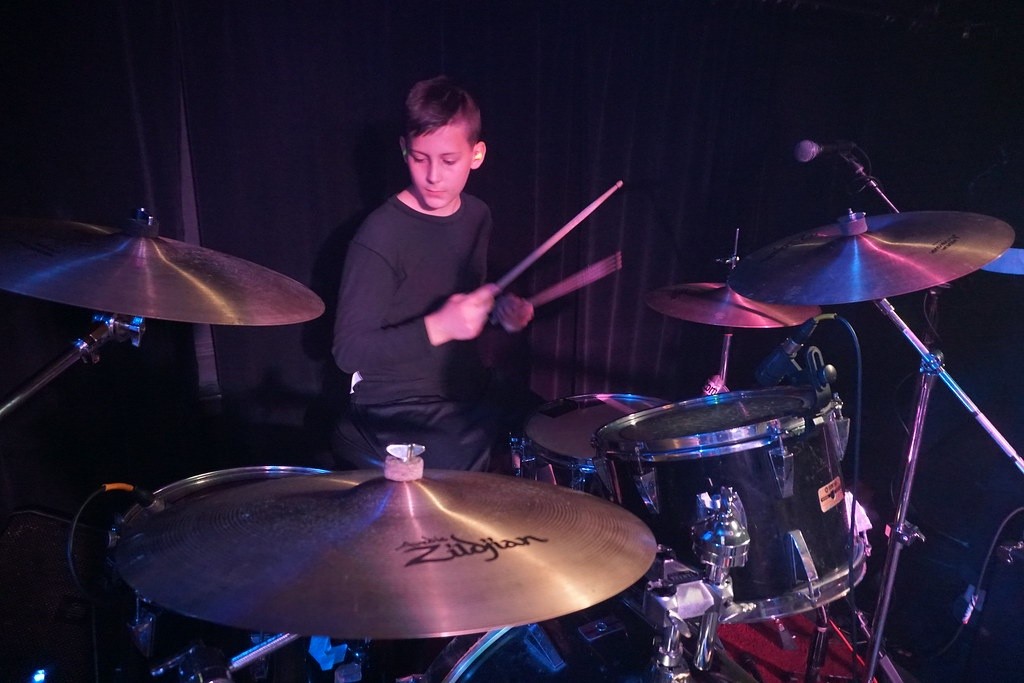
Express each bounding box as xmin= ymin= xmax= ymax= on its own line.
xmin=131 ymin=485 xmax=166 ymax=513
xmin=794 ymin=139 xmax=854 ymax=163
xmin=753 ymin=316 xmax=819 ymax=386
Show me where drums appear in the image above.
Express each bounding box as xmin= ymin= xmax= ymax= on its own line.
xmin=102 ymin=467 xmax=335 ymax=677
xmin=506 ymin=389 xmax=678 ymax=501
xmin=422 ymin=599 xmax=666 ymax=682
xmin=587 ymin=380 xmax=870 ymax=626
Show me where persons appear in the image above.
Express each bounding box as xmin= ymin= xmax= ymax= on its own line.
xmin=331 ymin=78 xmax=534 ymax=469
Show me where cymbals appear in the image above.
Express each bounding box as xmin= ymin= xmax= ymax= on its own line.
xmin=644 ymin=281 xmax=824 ymax=329
xmin=113 ymin=462 xmax=656 ymax=638
xmin=728 ymin=209 xmax=1016 ymax=308
xmin=0 ymin=234 xmax=328 ymax=329
